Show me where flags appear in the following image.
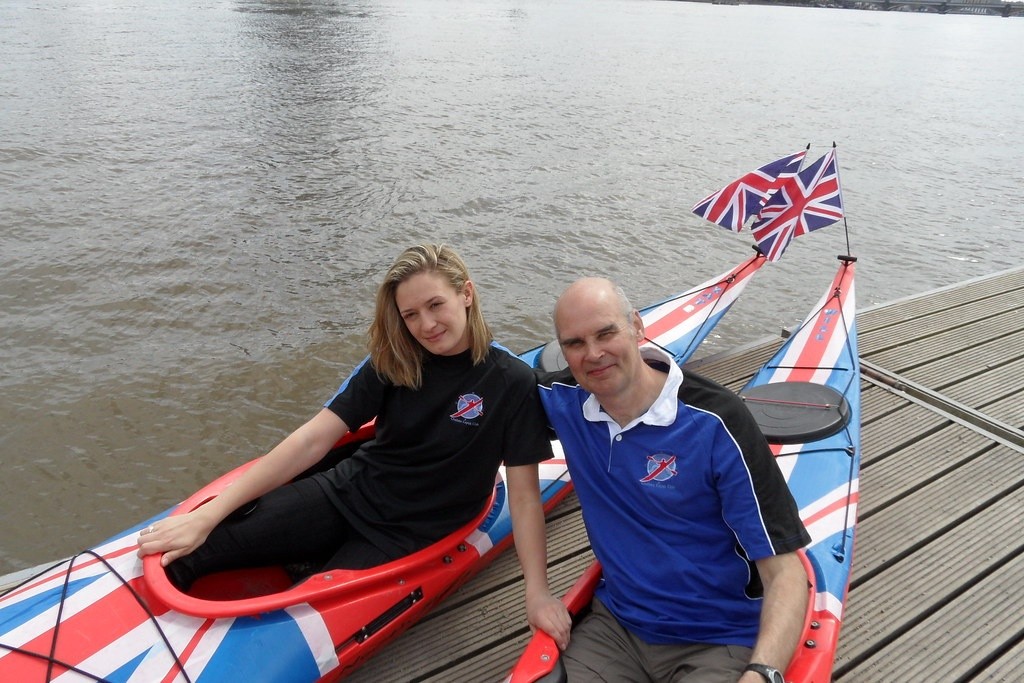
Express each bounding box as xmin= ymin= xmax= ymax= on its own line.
xmin=750 ymin=149 xmax=843 ymax=262
xmin=691 ymin=151 xmax=807 ymax=232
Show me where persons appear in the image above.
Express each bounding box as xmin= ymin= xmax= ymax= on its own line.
xmin=138 ymin=244 xmax=573 ymax=650
xmin=531 ymin=276 xmax=812 ymax=683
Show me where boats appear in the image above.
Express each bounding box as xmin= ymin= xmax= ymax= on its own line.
xmin=0 ymin=241 xmax=764 ymax=683
xmin=499 ymin=251 xmax=857 ymax=683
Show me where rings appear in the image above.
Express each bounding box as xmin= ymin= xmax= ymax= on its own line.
xmin=148 ymin=526 xmax=153 ymax=532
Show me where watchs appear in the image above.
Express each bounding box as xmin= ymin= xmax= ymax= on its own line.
xmin=744 ymin=664 xmax=786 ymax=683
